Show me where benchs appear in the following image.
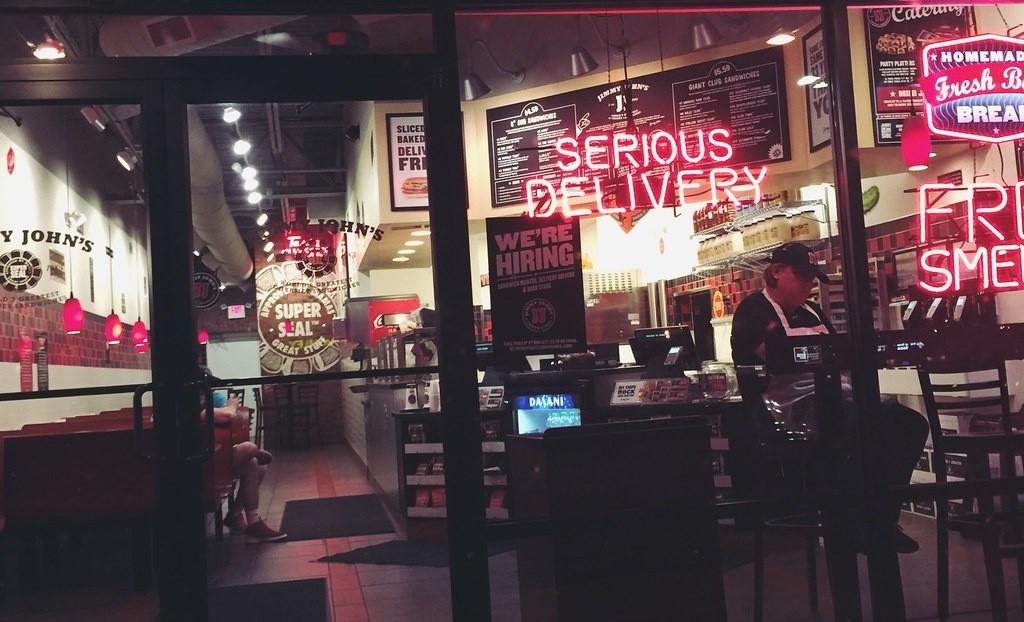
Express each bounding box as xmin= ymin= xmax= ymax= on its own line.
xmin=0 ymin=403 xmax=253 ymax=622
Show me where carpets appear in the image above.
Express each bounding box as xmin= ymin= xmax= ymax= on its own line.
xmin=277 ymin=494 xmax=396 ymax=542
xmin=208 ymin=578 xmax=328 ymax=622
xmin=307 ymin=540 xmax=517 ymax=568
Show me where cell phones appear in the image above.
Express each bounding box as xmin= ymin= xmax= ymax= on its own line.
xmin=233 ymin=390 xmax=240 ymax=399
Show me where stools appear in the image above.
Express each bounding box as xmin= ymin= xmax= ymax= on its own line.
xmin=227 ymin=388 xmax=244 ymax=409
xmin=252 ymin=384 xmax=324 ymax=449
xmin=734 ymin=370 xmax=907 ymax=622
xmin=901 ymin=294 xmax=1024 ymax=622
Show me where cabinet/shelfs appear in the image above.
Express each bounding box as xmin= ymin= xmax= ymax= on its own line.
xmin=394 ymin=412 xmax=516 ymax=541
xmin=689 ymin=198 xmax=831 ymax=284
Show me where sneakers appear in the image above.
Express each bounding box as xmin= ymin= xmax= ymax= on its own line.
xmin=245 ymin=518 xmax=288 ymax=543
xmin=222 ymin=512 xmax=248 ymax=534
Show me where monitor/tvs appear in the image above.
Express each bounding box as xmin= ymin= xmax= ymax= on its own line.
xmin=634 ymin=326 xmax=703 ymax=369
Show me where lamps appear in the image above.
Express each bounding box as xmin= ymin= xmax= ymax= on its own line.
xmin=344 ymin=124 xmax=359 ymax=143
xmin=570 ymin=12 xmax=630 ymax=77
xmin=33 ymin=40 xmax=274 ymax=355
xmin=765 ymin=13 xmax=796 ymax=46
xmin=351 ymin=343 xmax=364 ymax=363
xmin=286 ymin=319 xmax=291 ymax=333
xmin=465 ymin=38 xmax=525 ymax=100
xmin=691 ymin=12 xmax=749 ymax=51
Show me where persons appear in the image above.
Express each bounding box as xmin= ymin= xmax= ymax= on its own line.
xmin=198 ymin=365 xmax=288 ymax=544
xmin=731 ymin=243 xmax=928 ymax=552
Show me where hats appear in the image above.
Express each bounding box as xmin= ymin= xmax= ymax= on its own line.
xmin=767 ymin=242 xmax=829 ymax=284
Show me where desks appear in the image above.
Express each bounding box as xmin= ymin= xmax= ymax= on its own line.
xmin=266 ymin=382 xmax=297 ymax=442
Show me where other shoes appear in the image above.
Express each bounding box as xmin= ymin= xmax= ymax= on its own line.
xmin=831 ymin=503 xmax=919 ymax=557
